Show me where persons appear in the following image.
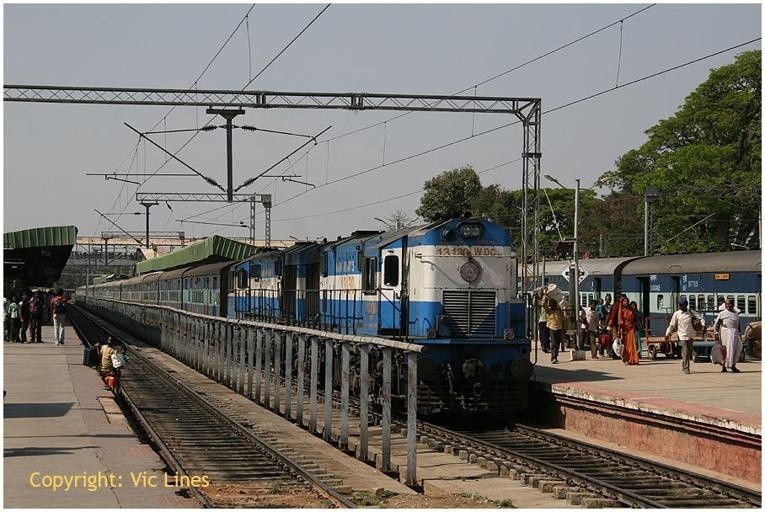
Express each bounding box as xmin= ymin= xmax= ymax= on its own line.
xmin=535 ymin=288 xmax=743 ymax=374
xmin=95 ymin=335 xmax=127 ymax=395
xmin=3 ymin=286 xmax=67 ymax=346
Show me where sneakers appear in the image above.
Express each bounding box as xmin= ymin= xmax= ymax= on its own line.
xmin=4 ymin=337 xmax=44 ymax=343
xmin=681 ymin=367 xmax=690 ymax=374
xmin=722 ymin=367 xmax=727 ymax=372
xmin=542 ymin=347 xmax=559 ymax=364
xmin=592 ymin=353 xmax=612 ymax=359
xmin=731 ymin=366 xmax=739 ymax=372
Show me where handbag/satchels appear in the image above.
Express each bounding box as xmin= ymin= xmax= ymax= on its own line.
xmin=111 ymin=352 xmax=126 ymax=369
xmin=612 ymin=337 xmax=625 ymax=358
xmin=710 ymin=343 xmax=726 ymax=366
xmin=692 ymin=316 xmax=702 ymax=331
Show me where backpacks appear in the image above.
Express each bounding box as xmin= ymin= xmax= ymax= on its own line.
xmin=28 ymin=294 xmax=42 ymax=315
xmin=54 ymin=296 xmax=66 ymax=314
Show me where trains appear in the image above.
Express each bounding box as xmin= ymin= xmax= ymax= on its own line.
xmin=518 ymin=249 xmax=761 ymax=360
xmin=72 ymin=212 xmax=534 ymax=419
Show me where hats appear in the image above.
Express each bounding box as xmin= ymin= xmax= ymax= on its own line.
xmin=676 ymin=300 xmax=687 ymax=305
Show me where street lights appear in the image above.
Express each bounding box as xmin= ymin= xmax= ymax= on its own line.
xmin=288 ymin=234 xmax=325 ymax=244
xmin=543 ymin=174 xmax=611 ymax=360
xmin=373 ymin=215 xmax=419 ymax=230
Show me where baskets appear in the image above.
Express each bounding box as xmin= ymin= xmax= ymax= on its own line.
xmin=83 ymin=346 xmax=102 ymax=366
